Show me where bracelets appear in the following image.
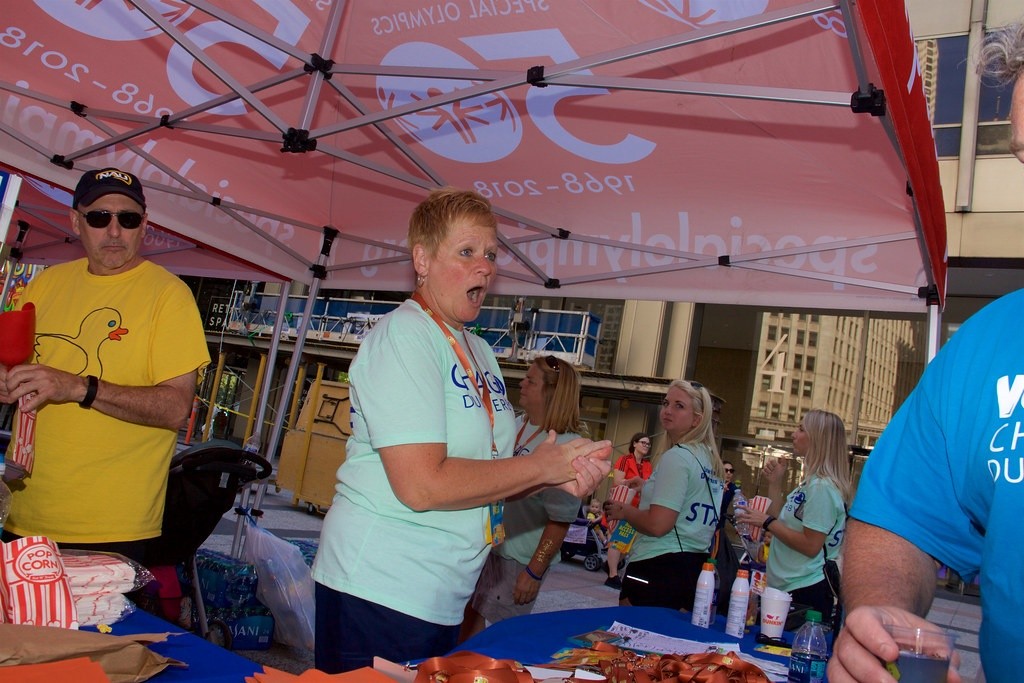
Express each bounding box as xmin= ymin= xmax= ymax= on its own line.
xmin=762 ymin=515 xmax=777 ymax=532
xmin=78 ymin=375 xmax=98 ymax=409
xmin=525 ymin=565 xmax=543 ymax=580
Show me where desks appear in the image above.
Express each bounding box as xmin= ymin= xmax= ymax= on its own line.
xmin=296 ymin=379 xmax=354 ymax=441
xmin=401 ymin=605 xmax=836 ymax=683
xmin=80 ymin=605 xmax=264 ymax=683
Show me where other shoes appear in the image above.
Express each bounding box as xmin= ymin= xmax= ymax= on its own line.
xmin=601 ymin=560 xmax=610 ymax=577
xmin=604 ymin=575 xmax=621 ymax=590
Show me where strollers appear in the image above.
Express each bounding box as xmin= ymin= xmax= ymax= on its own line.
xmin=135 ymin=438 xmax=273 ymax=651
xmin=729 ymin=513 xmax=767 ymax=585
xmin=561 ymin=505 xmax=626 ymax=572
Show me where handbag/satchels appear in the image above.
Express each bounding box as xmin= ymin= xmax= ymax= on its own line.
xmin=245 ymin=524 xmax=316 ymax=652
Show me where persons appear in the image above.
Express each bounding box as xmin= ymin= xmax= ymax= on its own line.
xmin=826 ymin=21 xmax=1024 ymax=683
xmin=733 ymin=409 xmax=854 ymax=633
xmin=602 ymin=380 xmax=725 ymax=614
xmin=604 ymin=433 xmax=652 ymax=590
xmin=708 ymin=461 xmax=737 ymax=558
xmin=586 ymin=499 xmax=607 ymax=547
xmin=311 ymin=187 xmax=614 ymax=675
xmin=457 ymin=354 xmax=590 ymax=646
xmin=0 ymin=166 xmax=212 ymax=557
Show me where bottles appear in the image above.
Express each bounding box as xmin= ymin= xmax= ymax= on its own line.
xmin=708 ymin=558 xmax=721 ymax=625
xmin=179 ymin=538 xmax=319 ymax=648
xmin=692 ymin=562 xmax=715 ymax=629
xmin=733 ymin=488 xmax=751 ymax=534
xmin=787 ymin=610 xmax=828 ymax=683
xmin=726 ymin=569 xmax=750 ymax=639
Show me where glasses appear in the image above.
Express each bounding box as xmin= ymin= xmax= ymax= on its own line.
xmin=725 ymin=468 xmax=734 ymax=473
xmin=691 ymin=381 xmax=703 ymax=388
xmin=545 ymin=355 xmax=559 ymax=370
xmin=638 ymin=441 xmax=651 ymax=448
xmin=77 ymin=207 xmax=144 ymax=229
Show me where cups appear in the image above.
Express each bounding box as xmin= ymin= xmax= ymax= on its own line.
xmin=881 ymin=623 xmax=956 ymax=683
xmin=759 ymin=586 xmax=792 ymax=641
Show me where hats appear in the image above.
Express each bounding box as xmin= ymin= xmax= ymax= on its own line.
xmin=73 ymin=167 xmax=146 ymax=209
